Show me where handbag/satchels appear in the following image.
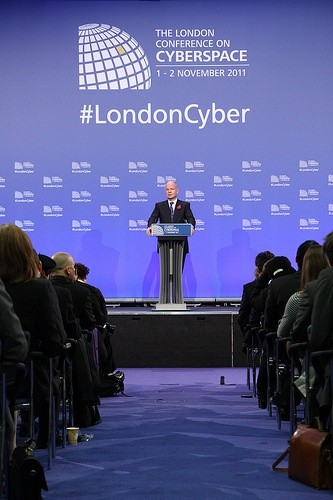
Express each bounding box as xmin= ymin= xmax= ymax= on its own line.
xmin=272 ymin=425 xmax=333 ymax=490
xmin=6 ymin=459 xmax=48 ymax=500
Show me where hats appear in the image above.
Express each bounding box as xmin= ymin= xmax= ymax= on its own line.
xmin=36 ymin=254 xmax=56 ymax=272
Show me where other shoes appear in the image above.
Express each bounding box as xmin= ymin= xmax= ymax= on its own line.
xmin=115 ymin=370 xmax=124 ymax=380
xmin=18 ymin=439 xmax=36 ymax=459
xmin=258 ymin=400 xmax=267 ymax=409
xmin=79 ymin=409 xmax=103 ymax=427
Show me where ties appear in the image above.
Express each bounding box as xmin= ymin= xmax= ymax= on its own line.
xmin=169 ymin=202 xmax=174 ymax=223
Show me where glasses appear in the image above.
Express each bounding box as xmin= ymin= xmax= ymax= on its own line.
xmin=68 ymin=266 xmax=76 ymax=271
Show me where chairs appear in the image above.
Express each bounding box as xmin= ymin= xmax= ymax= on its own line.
xmin=242 ymin=324 xmax=333 ymax=443
xmin=0 ymin=323 xmax=115 ymax=471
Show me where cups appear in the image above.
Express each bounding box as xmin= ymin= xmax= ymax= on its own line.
xmin=67 ymin=427 xmax=79 ymax=445
